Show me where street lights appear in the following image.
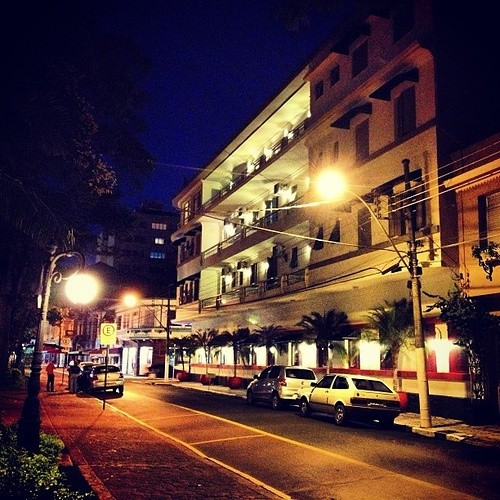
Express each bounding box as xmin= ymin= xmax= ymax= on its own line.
xmin=313 ymin=166 xmax=434 ymax=428
xmin=12 ymin=250 xmax=102 ymax=451
xmin=124 ymin=294 xmax=171 ymax=384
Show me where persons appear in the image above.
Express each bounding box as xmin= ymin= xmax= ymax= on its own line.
xmin=67 ymin=360 xmax=84 ymax=394
xmin=45 ymin=359 xmax=58 ymax=393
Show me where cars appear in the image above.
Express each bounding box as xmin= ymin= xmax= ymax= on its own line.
xmin=80 ymin=364 xmax=124 ymax=394
xmin=244 ymin=366 xmax=319 ymax=409
xmin=302 ymin=374 xmax=403 ymax=427
